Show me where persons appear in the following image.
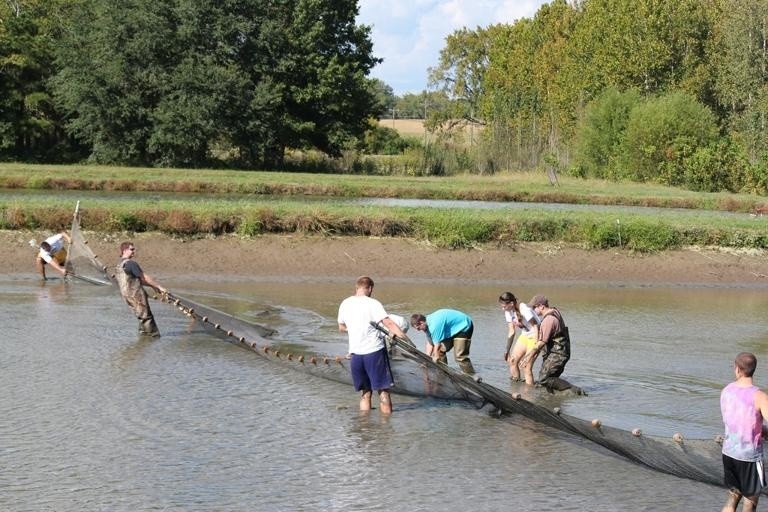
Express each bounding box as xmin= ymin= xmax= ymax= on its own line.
xmin=36 ymin=231 xmax=72 ymax=281
xmin=411 ymin=308 xmax=475 ymax=386
xmin=719 ymin=352 xmax=768 ymax=512
xmin=114 ymin=241 xmax=163 ymax=339
xmin=497 ymin=291 xmax=541 ymax=384
xmin=518 ymin=294 xmax=588 ymax=396
xmin=336 ymin=276 xmax=403 ymax=414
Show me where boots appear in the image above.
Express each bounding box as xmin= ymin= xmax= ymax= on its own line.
xmin=454 ymin=338 xmax=475 ymax=375
xmin=438 ymin=341 xmax=448 ymax=365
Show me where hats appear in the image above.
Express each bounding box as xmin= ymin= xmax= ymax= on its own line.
xmin=527 ymin=295 xmax=548 ymax=306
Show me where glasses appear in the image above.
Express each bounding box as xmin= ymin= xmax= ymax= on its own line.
xmin=129 ymin=248 xmax=135 ymax=251
